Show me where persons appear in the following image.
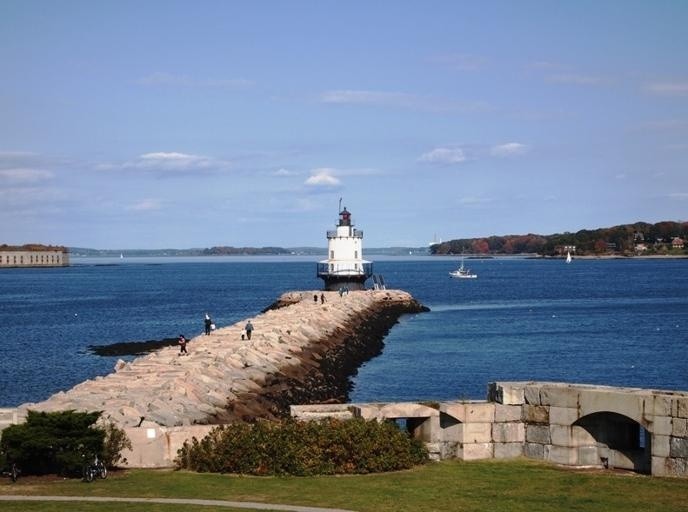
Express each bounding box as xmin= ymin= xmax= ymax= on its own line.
xmin=178 ymin=335 xmax=188 ymax=354
xmin=321 ymin=293 xmax=327 ymax=305
xmin=240 ymin=328 xmax=246 ymax=340
xmin=245 ymin=320 xmax=254 ymax=339
xmin=313 ymin=294 xmax=318 ymax=303
xmin=204 ymin=314 xmax=212 ymax=335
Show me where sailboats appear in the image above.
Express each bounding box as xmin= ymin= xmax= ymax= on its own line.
xmin=449 ymin=246 xmax=479 ymax=279
xmin=566 ymin=251 xmax=573 ymax=264
